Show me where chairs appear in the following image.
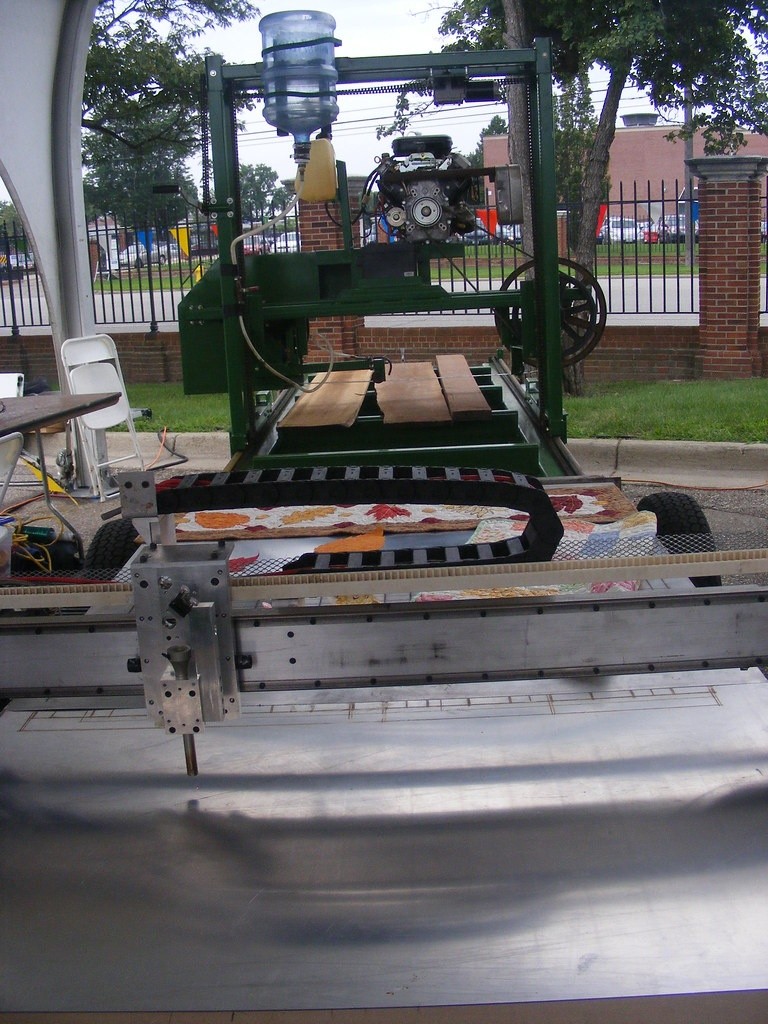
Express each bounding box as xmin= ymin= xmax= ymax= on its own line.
xmin=0 ymin=372 xmax=26 ymax=517
xmin=60 ymin=335 xmax=145 ymax=505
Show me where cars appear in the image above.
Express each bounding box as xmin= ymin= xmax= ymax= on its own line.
xmin=657 ymin=214 xmax=699 ymax=242
xmin=493 ymin=221 xmax=521 ymax=244
xmin=170 ymin=244 xmax=181 ymax=256
xmin=269 ymin=232 xmax=302 ymax=254
xmin=119 ymin=244 xmax=171 ymax=268
xmin=10 ymin=253 xmax=35 ymax=269
xmin=190 ymin=242 xmax=219 ymax=256
xmin=761 ymin=219 xmax=767 ymax=241
xmin=600 ymin=217 xmax=644 ymax=243
xmin=455 ymin=218 xmax=491 ymax=245
xmin=243 ymin=235 xmax=270 ymax=254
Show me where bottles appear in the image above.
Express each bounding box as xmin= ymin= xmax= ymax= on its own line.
xmin=258 ymin=10 xmax=339 ymax=157
xmin=20 ymin=526 xmax=75 ymax=542
xmin=476 ymin=218 xmax=485 ymax=236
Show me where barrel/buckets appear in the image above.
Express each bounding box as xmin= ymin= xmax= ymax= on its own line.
xmin=0 ymin=517 xmax=15 ymax=579
xmin=476 ymin=209 xmax=497 ymax=235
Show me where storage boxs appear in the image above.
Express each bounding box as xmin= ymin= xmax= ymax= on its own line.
xmin=0 ymin=524 xmax=16 ymax=577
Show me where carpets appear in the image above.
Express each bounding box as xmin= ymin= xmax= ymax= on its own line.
xmin=134 ymin=476 xmax=658 ymax=605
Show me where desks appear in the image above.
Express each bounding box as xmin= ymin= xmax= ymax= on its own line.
xmin=0 ymin=391 xmax=121 ymax=565
